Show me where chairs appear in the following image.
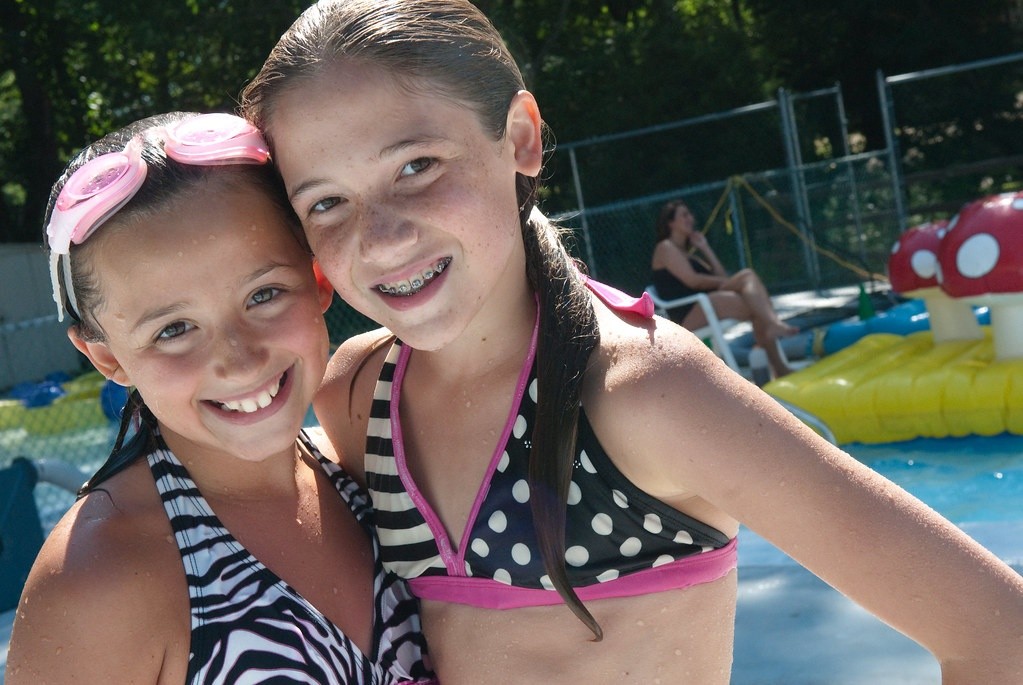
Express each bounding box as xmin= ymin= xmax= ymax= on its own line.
xmin=646 ymin=284 xmax=788 ymax=391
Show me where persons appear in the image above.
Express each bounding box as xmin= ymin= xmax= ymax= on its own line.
xmin=0 ymin=111 xmax=445 ymax=685
xmin=243 ymin=0 xmax=1023 ymax=685
xmin=649 ymin=198 xmax=805 ymax=378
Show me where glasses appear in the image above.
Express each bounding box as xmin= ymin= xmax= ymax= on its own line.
xmin=48 ymin=111 xmax=270 ymax=255
